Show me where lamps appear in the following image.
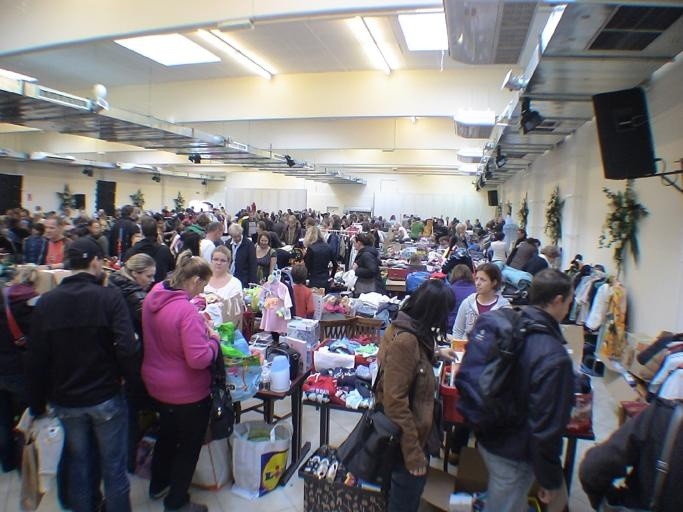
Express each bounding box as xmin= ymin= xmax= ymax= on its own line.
xmin=496 ymin=66 xmax=530 ymax=94
xmin=79 ymin=133 xmax=298 ymax=186
xmin=90 ymin=95 xmax=112 ymax=113
xmin=471 ymin=98 xmax=545 ymax=194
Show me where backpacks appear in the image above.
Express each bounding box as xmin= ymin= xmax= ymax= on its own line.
xmin=453 ymin=305 xmax=553 ymax=444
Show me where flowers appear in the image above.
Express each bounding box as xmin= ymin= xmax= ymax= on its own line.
xmin=53 ymin=182 xmax=186 ymax=212
xmin=490 ymin=177 xmax=571 ymax=249
xmin=594 ymin=174 xmax=651 ymax=283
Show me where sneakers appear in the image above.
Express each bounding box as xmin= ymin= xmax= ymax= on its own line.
xmin=149 ymin=481 xmax=211 ymax=511
xmin=3 ymin=451 xmax=22 ymax=479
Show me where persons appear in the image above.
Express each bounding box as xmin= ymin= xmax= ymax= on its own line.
xmin=0 ymin=200 xmax=574 ymax=511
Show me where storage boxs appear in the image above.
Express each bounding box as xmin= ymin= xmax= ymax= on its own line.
xmin=593 ymin=329 xmax=677 ymax=432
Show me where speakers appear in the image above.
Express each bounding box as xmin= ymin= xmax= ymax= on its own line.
xmin=592 ymin=87 xmax=656 ymax=179
xmin=488 ymin=190 xmax=499 ymax=206
xmin=73 ymin=194 xmax=85 ymax=209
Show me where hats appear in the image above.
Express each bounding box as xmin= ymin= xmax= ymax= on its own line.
xmin=67 ymin=234 xmax=105 ymax=262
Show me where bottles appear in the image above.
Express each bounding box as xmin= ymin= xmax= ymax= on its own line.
xmin=259 ymin=358 xmax=271 ymax=391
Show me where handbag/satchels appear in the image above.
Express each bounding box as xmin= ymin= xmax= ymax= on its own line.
xmin=328 ymin=328 xmax=423 ymax=491
xmin=200 ymin=336 xmax=238 ymax=449
xmin=353 ymin=252 xmax=381 ymax=298
xmin=189 ymin=423 xmax=231 ymax=490
xmin=232 ymin=420 xmax=294 ymax=502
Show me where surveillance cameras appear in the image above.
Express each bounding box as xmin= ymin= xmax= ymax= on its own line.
xmin=501 ymin=70 xmax=512 ymax=90
